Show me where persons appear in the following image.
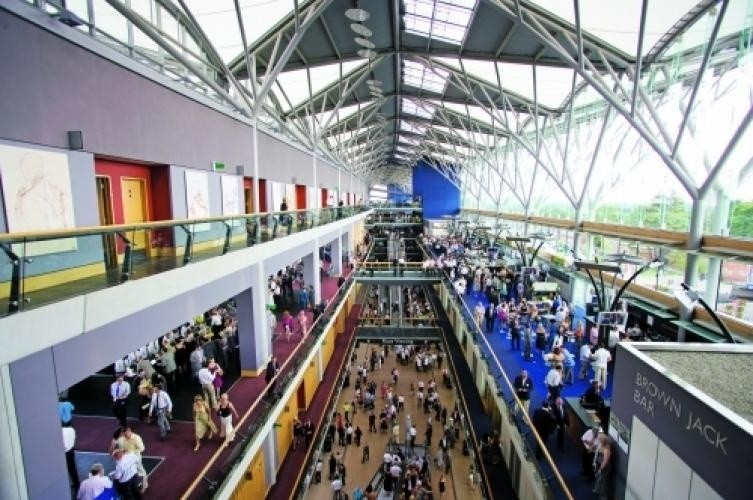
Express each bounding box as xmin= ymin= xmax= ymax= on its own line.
xmin=279 ymin=198 xmax=289 ymax=223
xmin=267 ymin=242 xmax=346 ymax=343
xmin=364 ymin=284 xmax=438 ymax=327
xmin=336 ymin=200 xmax=343 ymax=221
xmin=420 ymin=230 xmax=528 ymax=303
xmin=290 ymin=341 xmax=486 ymax=500
xmin=470 ymin=292 xmax=644 ymax=500
xmin=361 ymin=201 xmax=422 ymax=245
xmin=57 ymin=297 xmax=280 ymax=500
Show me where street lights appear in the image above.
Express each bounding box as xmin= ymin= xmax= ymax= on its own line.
xmin=671 ymin=274 xmax=738 ymax=344
xmin=604 ymin=254 xmax=670 ymax=352
xmin=447 ymin=217 xmax=559 ymax=292
